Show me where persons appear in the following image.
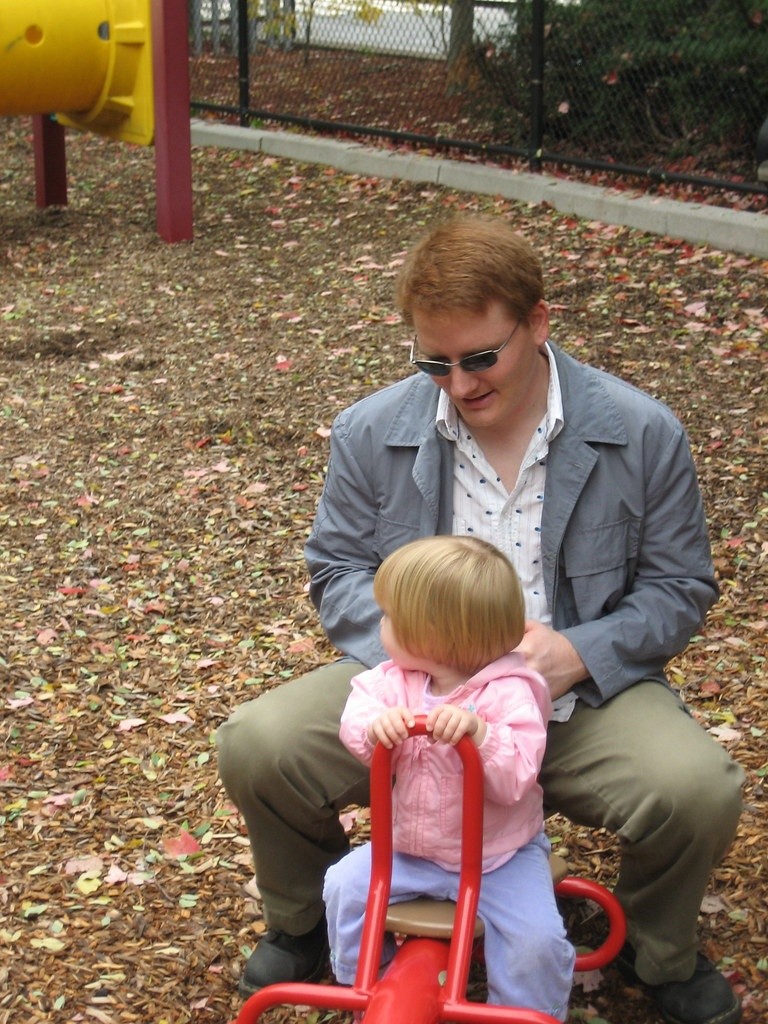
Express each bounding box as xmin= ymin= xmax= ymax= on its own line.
xmin=323 ymin=537 xmax=577 ymax=1024
xmin=216 ymin=223 xmax=747 ymax=1024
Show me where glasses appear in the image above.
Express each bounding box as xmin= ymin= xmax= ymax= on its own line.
xmin=410 ymin=316 xmax=525 ymax=377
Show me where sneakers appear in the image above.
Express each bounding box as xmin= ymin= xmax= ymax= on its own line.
xmin=625 ymin=934 xmax=741 ymax=1024
xmin=237 ymin=911 xmax=330 ymax=1001
